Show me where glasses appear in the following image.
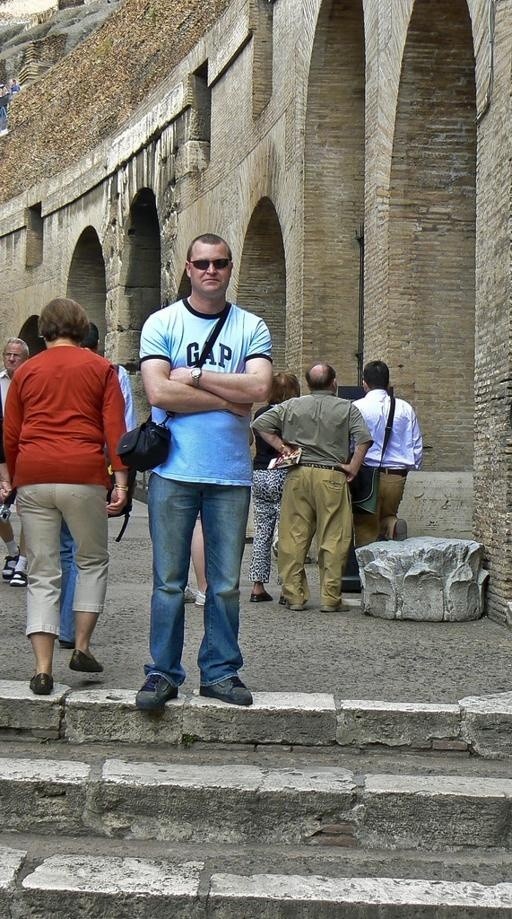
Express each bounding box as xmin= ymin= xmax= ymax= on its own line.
xmin=190 ymin=258 xmax=230 ymax=270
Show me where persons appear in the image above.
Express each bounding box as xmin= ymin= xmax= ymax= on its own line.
xmin=249 ymin=364 xmax=374 ymax=612
xmin=135 ymin=233 xmax=273 ymax=713
xmin=354 ymin=360 xmax=424 ymax=550
xmin=0 ymin=79 xmax=21 ymax=98
xmin=1 ymin=298 xmax=135 ymax=697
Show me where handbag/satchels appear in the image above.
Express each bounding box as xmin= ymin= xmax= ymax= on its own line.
xmin=106 ymin=466 xmax=137 ymax=542
xmin=115 ymin=421 xmax=171 ymax=473
xmin=345 ymin=453 xmax=380 ymax=515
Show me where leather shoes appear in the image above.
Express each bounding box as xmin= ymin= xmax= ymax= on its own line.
xmin=393 ymin=519 xmax=407 ymax=541
xmin=30 ymin=673 xmax=53 ymax=694
xmin=69 ymin=649 xmax=104 ymax=673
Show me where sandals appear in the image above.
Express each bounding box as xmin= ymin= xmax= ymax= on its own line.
xmin=2 ymin=545 xmax=20 ymax=580
xmin=9 ymin=571 xmax=28 ymax=587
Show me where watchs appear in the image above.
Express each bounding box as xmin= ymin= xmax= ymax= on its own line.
xmin=192 ymin=368 xmax=202 ymax=388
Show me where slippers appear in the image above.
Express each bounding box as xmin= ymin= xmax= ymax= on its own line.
xmin=250 ymin=591 xmax=273 ymax=602
xmin=279 ymin=596 xmax=286 ymax=605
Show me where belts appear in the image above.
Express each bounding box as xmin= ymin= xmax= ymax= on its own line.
xmin=379 ymin=466 xmax=408 ymax=477
xmin=298 ymin=463 xmax=349 ymax=477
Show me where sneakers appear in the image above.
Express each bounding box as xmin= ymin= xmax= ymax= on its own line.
xmin=194 ymin=590 xmax=206 ymax=606
xmin=320 ymin=602 xmax=343 ymax=612
xmin=284 ymin=599 xmax=304 ymax=611
xmin=183 ymin=584 xmax=198 ymax=603
xmin=200 ymin=676 xmax=252 ymax=705
xmin=136 ymin=675 xmax=179 ymax=711
